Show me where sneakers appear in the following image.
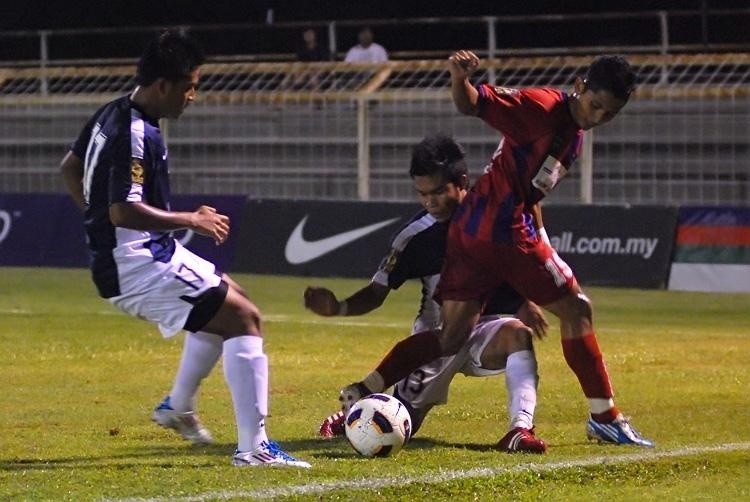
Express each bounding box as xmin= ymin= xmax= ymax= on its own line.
xmin=584 ymin=412 xmax=657 ymax=448
xmin=319 ymin=410 xmax=346 ymax=441
xmin=338 ymin=381 xmax=373 ymax=413
xmin=150 ymin=394 xmax=213 ymax=445
xmin=496 ymin=426 xmax=547 ymax=454
xmin=230 ymin=438 xmax=313 ymax=470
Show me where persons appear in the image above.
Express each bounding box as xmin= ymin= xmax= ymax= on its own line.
xmin=289 ymin=28 xmax=331 ymax=91
xmin=57 ymin=24 xmax=314 ymax=471
xmin=338 ymin=47 xmax=658 ymax=450
xmin=301 ymin=131 xmax=553 ymax=454
xmin=341 ymin=26 xmax=389 ymax=92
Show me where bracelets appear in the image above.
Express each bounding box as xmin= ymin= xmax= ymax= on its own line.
xmin=339 ymin=300 xmax=348 ymax=316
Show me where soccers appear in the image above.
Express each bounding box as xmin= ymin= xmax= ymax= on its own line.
xmin=345 ymin=393 xmax=413 ymax=458
